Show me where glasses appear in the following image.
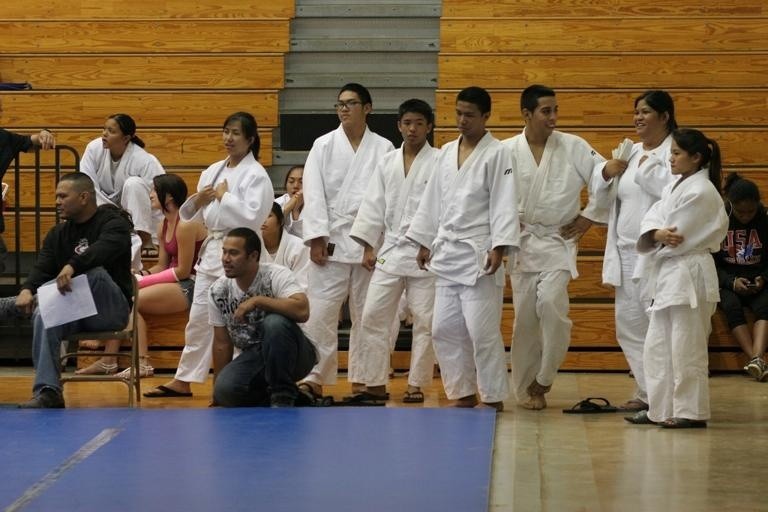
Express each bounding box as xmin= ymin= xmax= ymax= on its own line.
xmin=333 ymin=101 xmax=361 ymax=111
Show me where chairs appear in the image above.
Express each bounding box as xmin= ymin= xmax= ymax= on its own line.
xmin=60 ymin=273 xmax=141 ymax=407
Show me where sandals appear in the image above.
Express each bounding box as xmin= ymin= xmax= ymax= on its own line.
xmin=74 ymin=360 xmax=119 ymax=375
xmin=114 ymin=364 xmax=154 ymax=379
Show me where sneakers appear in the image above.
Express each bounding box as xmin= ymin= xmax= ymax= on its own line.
xmin=757 ymin=365 xmax=767 ymax=381
xmin=17 ymin=388 xmax=65 ymax=409
xmin=743 ymin=356 xmax=766 ymax=379
xmin=0 ymin=295 xmax=35 ymax=319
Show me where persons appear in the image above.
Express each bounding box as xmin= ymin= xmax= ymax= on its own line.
xmin=0 ymin=125 xmax=58 ymax=273
xmin=0 ymin=171 xmax=136 ymax=410
xmin=588 ymin=89 xmax=692 ymax=413
xmin=498 ymin=85 xmax=616 ymax=411
xmin=80 ymin=112 xmax=304 ymax=399
xmin=711 ymin=169 xmax=768 ymax=383
xmin=303 ymin=82 xmax=397 ymax=403
xmin=622 ymin=125 xmax=731 ymax=423
xmin=348 ymin=97 xmax=444 ymax=405
xmin=402 ymin=85 xmax=522 ymax=411
xmin=208 ymin=228 xmax=322 ymax=407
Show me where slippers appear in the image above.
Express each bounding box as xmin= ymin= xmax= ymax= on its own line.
xmin=297 ymin=383 xmax=322 ymax=398
xmin=617 ymin=399 xmax=648 ymax=411
xmin=623 ymin=409 xmax=657 ymax=423
xmin=143 ymin=385 xmax=193 ymax=397
xmin=658 ymin=417 xmax=706 ymax=427
xmin=562 ymin=398 xmax=617 ymax=413
xmin=342 ymin=391 xmax=389 ymax=401
xmin=402 ymin=391 xmax=424 ymax=402
xmin=141 ymin=247 xmax=158 ymax=258
